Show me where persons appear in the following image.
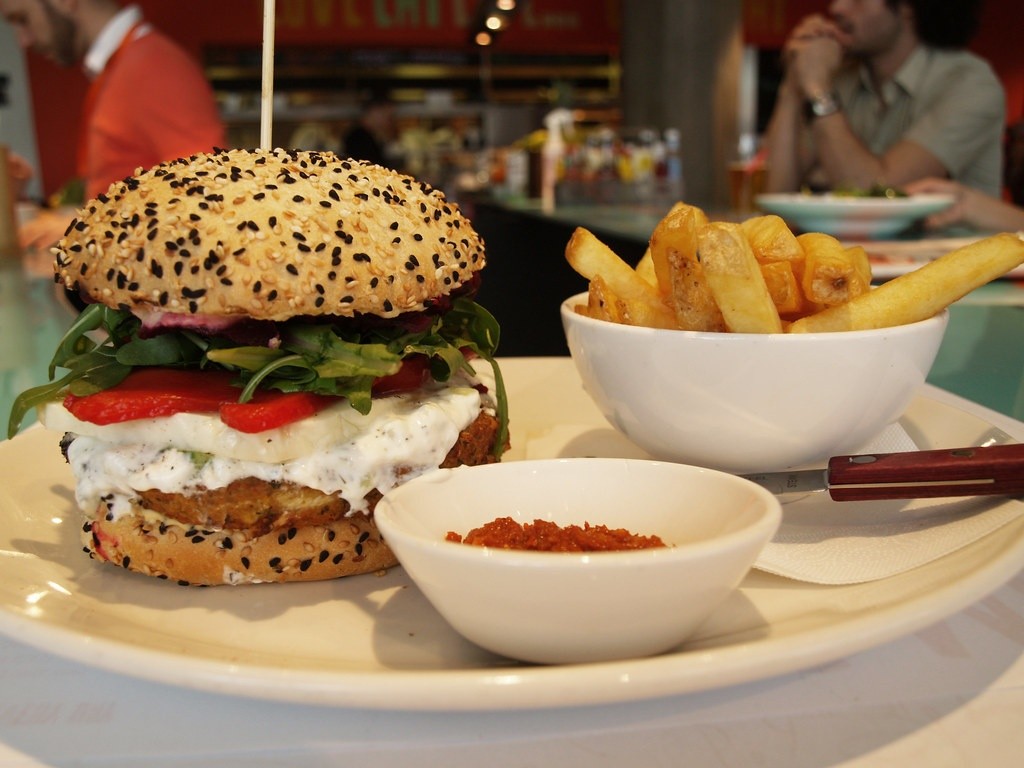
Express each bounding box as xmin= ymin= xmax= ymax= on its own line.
xmin=338 ymin=96 xmax=410 ymax=175
xmin=904 ymin=178 xmax=1024 ymax=235
xmin=769 ymin=1 xmax=1004 ymax=201
xmin=0 ymin=0 xmax=233 ymax=255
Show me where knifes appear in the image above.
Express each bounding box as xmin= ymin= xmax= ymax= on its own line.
xmin=739 ymin=444 xmax=1024 ymax=497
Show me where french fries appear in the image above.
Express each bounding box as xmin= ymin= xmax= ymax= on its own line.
xmin=563 ymin=200 xmax=1024 ymax=334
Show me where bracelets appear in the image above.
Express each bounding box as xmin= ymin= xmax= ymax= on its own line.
xmin=804 ymin=91 xmax=842 ymax=120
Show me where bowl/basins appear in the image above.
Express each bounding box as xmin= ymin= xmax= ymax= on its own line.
xmin=374 ymin=458 xmax=784 ymax=663
xmin=561 ymin=292 xmax=949 ymax=478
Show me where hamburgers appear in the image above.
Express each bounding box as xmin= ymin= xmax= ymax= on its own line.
xmin=4 ymin=146 xmax=510 ymax=585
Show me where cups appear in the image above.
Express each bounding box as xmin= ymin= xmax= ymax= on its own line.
xmin=725 ymin=134 xmax=768 ymax=214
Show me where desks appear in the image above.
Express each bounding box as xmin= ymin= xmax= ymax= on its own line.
xmin=474 ymin=198 xmax=1024 ymax=424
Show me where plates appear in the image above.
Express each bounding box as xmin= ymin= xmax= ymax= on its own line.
xmin=844 ymin=239 xmax=1024 ymax=279
xmin=753 ymin=195 xmax=953 ymax=237
xmin=0 ymin=356 xmax=1024 ymax=713
